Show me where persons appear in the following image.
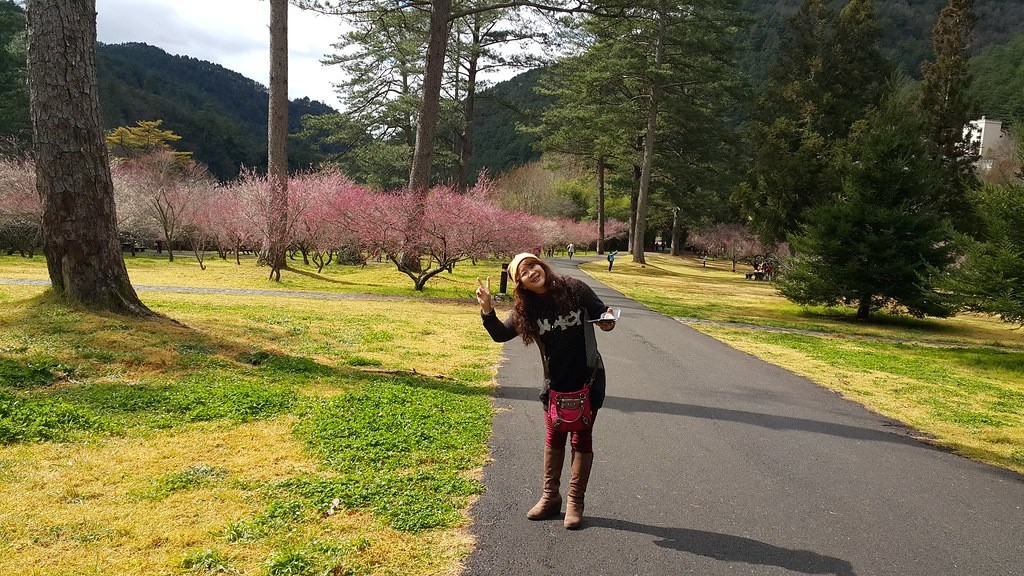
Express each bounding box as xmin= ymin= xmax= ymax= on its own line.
xmin=608 ymin=250 xmax=617 ymax=272
xmin=567 ymin=242 xmax=574 ymax=260
xmin=650 ymin=241 xmax=666 ymax=252
xmin=702 ymin=254 xmax=708 ymax=268
xmin=546 ymin=247 xmax=555 ymax=257
xmin=474 ymin=253 xmax=616 ymax=531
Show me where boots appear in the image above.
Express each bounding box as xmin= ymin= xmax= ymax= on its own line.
xmin=563 ymin=449 xmax=594 ymax=529
xmin=527 ymin=446 xmax=566 ymax=519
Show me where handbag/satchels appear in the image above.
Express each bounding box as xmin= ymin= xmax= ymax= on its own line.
xmin=549 ymin=388 xmax=592 ymax=432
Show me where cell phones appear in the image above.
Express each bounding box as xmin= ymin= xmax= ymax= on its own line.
xmin=586 ymin=318 xmax=613 ymax=323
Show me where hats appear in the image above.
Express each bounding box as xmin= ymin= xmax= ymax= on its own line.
xmin=508 ymin=253 xmax=541 ymax=283
